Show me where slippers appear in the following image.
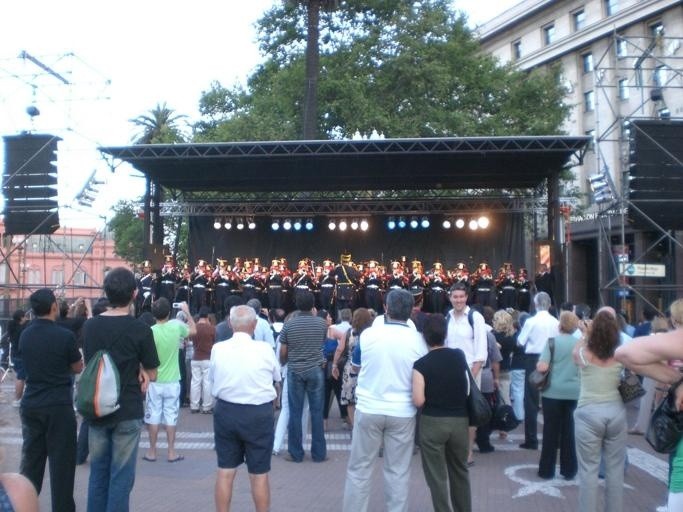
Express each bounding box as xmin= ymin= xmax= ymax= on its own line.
xmin=168 ymin=454 xmax=186 ymax=464
xmin=143 ymin=453 xmax=157 ymax=462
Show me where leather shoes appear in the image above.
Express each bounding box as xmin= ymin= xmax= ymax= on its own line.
xmin=203 ymin=410 xmax=214 ymax=414
xmin=190 ymin=408 xmax=199 ymax=412
xmin=480 ymin=446 xmax=496 ymax=452
xmin=313 ymin=457 xmax=330 ymax=462
xmin=520 ymin=441 xmax=538 ymax=450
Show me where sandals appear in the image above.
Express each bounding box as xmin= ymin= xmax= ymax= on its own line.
xmin=467 ymin=459 xmax=479 ymax=469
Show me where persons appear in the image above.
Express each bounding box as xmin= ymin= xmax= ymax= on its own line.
xmin=59 ymin=297 xmax=92 ymax=350
xmin=499 ymin=265 xmax=517 ymax=310
xmin=452 ymin=263 xmax=469 ymax=306
xmin=238 ymin=262 xmax=255 ymax=305
xmin=134 ymin=260 xmax=158 ymax=319
xmin=411 ymin=313 xmax=471 ymax=512
xmin=315 ymin=309 xmax=347 ymax=433
xmin=427 ymin=263 xmax=446 ymax=316
xmin=272 ymin=308 xmax=285 ymax=409
xmin=593 ymin=306 xmax=632 ymax=480
xmin=341 ymin=289 xmax=428 ymax=512
xmin=18 ymin=289 xmax=82 ymax=512
xmin=317 ymin=261 xmax=338 ymax=314
xmin=534 ymin=264 xmax=554 ymax=305
xmin=469 ymin=303 xmax=503 ymax=454
xmin=509 ymin=313 xmax=532 ymax=423
xmin=189 ymin=306 xmax=215 ymax=414
xmin=212 ymin=259 xmax=237 ymax=323
xmin=141 ymin=297 xmax=196 ymax=462
xmin=215 ymin=295 xmax=254 ymax=343
xmin=271 ymin=310 xmax=308 ymax=457
xmin=627 ymin=315 xmax=668 ymax=435
xmin=331 ymin=308 xmax=372 ymax=427
xmin=407 ymin=261 xmax=427 ymax=311
xmin=476 ymin=263 xmax=492 ymax=307
xmin=516 ymin=291 xmax=562 ymax=450
xmin=654 ymin=299 xmax=682 ymax=491
xmin=513 ymin=269 xmax=531 ymax=308
xmin=334 ymin=309 xmax=352 ymax=344
xmin=7 ymin=310 xmax=25 ymax=407
xmin=535 ymin=311 xmax=581 ymax=481
xmin=174 ymin=264 xmax=191 ymax=314
xmin=444 ymin=283 xmax=488 ymax=469
xmin=207 ymin=305 xmax=281 ymax=512
xmin=612 ymin=329 xmax=682 ymax=512
xmin=489 ymin=310 xmax=516 ymax=444
xmin=387 ymin=262 xmax=406 ymax=290
xmin=363 ymin=261 xmax=383 ymax=315
xmin=246 ymin=298 xmax=275 ymax=347
xmin=0 ymin=474 xmax=38 ymax=512
xmin=327 ymin=252 xmax=361 ymax=323
xmin=278 ymin=291 xmax=329 ymax=463
xmin=571 ymin=311 xmax=627 ymax=512
xmin=189 ymin=259 xmax=212 ymax=319
xmin=631 ymin=308 xmax=653 ymax=409
xmin=157 ymin=256 xmax=179 ymax=321
xmin=82 ymin=267 xmax=160 ymax=512
xmin=175 ymin=311 xmax=189 ymax=407
xmin=290 ymin=261 xmax=318 ymax=310
xmin=263 ymin=260 xmax=282 ymax=311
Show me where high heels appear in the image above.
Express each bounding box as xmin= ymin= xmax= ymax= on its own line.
xmin=272 ymin=450 xmax=281 ymax=455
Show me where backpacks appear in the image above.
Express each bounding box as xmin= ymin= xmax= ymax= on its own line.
xmin=74 ymin=318 xmax=141 ymax=418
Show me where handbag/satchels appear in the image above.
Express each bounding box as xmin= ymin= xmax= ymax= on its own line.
xmin=493 ymin=385 xmax=520 ymax=432
xmin=529 ymin=337 xmax=554 ymax=391
xmin=618 ymin=368 xmax=647 ymax=403
xmin=454 ymin=348 xmax=492 ymax=428
xmin=336 ymin=327 xmax=350 ymax=370
xmin=327 ymin=327 xmax=333 ymax=386
xmin=645 ymin=376 xmax=683 ymax=454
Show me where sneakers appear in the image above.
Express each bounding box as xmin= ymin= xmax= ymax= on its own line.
xmin=497 ymin=436 xmax=512 ymax=445
xmin=342 ymin=423 xmax=354 ymax=429
xmin=283 ymin=453 xmax=298 ymax=463
xmin=628 ymin=427 xmax=643 ymax=435
xmin=13 ymin=400 xmax=20 ymax=406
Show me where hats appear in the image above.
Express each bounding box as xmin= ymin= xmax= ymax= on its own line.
xmin=504 ymin=263 xmax=512 ymax=272
xmin=227 ymin=265 xmax=231 ymax=272
xmin=281 ymin=258 xmax=286 ymax=264
xmin=218 ymin=260 xmax=227 ymax=267
xmin=199 ymin=260 xmax=207 ymax=266
xmin=323 ymin=260 xmax=332 ymax=266
xmin=401 ymin=255 xmax=407 ymax=262
xmin=480 ymin=263 xmax=488 ymax=271
xmin=164 ymin=255 xmax=173 ymax=263
xmin=254 ymin=265 xmax=259 ymax=272
xmin=184 ymin=263 xmax=190 ymax=270
xmin=304 ymin=258 xmax=309 ymax=265
xmin=316 ymin=266 xmax=323 ymax=273
xmin=358 ymin=264 xmax=364 ymax=271
xmin=299 ymin=261 xmax=307 ymax=268
xmin=254 ymin=258 xmax=260 ymax=263
xmin=262 ymin=267 xmax=268 ymax=273
xmin=500 ymin=267 xmax=505 ymax=272
xmin=411 ymin=290 xmax=423 ymax=296
xmin=519 ymin=267 xmax=528 ymax=276
xmin=412 ymin=261 xmax=421 ymax=268
xmin=272 ymin=260 xmax=281 ymax=267
xmin=370 ymin=260 xmax=378 ymax=268
xmin=341 ymin=253 xmax=351 ymax=262
xmin=235 ymin=257 xmax=240 ymax=263
xmin=458 ymin=263 xmax=465 ymax=270
xmin=143 ymin=260 xmax=152 ymax=267
xmin=392 ymin=261 xmax=401 ymax=269
xmin=435 ymin=263 xmax=442 ymax=270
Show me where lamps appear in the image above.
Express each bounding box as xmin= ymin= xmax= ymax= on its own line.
xmin=294 ymin=219 xmax=302 ymax=230
xmin=591 ymin=183 xmax=609 ymax=193
xmin=594 ymin=187 xmax=614 ymax=205
xmin=361 ymin=219 xmax=369 ymax=232
xmin=422 ymin=216 xmax=430 ymax=228
xmin=351 ymin=218 xmax=359 ymax=231
xmin=587 ymin=174 xmax=604 ymax=184
xmin=329 ymin=219 xmax=336 ymax=231
xmin=479 ymin=217 xmax=490 ymax=230
xmin=340 ymin=219 xmax=347 ymax=232
xmin=272 ymin=220 xmax=279 ymax=230
xmin=214 ymin=218 xmax=221 ymax=230
xmin=284 ymin=219 xmax=291 ymax=230
xmin=249 ymin=218 xmax=257 ymax=230
xmin=443 ymin=221 xmax=452 ymax=229
xmin=470 ymin=218 xmax=479 ymax=231
xmin=225 ymin=217 xmax=232 ymax=230
xmin=238 ymin=218 xmax=244 ymax=230
xmin=306 ymin=219 xmax=314 ymax=230
xmin=399 ymin=217 xmax=406 ymax=228
xmin=456 ymin=220 xmax=466 ymax=229
xmin=388 ymin=218 xmax=395 ymax=230
xmin=411 ymin=216 xmax=419 ymax=229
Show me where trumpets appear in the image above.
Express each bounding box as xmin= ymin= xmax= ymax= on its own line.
xmin=369 ymin=272 xmax=376 ymax=279
xmin=487 ymin=273 xmax=493 ymax=279
xmin=393 ymin=271 xmax=399 ymax=278
xmin=301 ymin=268 xmax=306 ymax=274
xmin=323 ymin=269 xmax=328 ymax=274
xmin=511 ymin=277 xmax=515 ymax=283
xmin=458 ymin=273 xmax=462 ymax=280
xmin=413 ymin=270 xmax=419 ymax=277
xmin=434 ymin=272 xmax=439 ymax=278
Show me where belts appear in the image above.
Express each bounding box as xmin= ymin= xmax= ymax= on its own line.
xmin=193 ymin=284 xmax=206 ymax=289
xmin=255 ymin=287 xmax=262 ymax=291
xmin=337 ymin=283 xmax=356 ymax=285
xmin=411 ymin=286 xmax=425 ymax=290
xmin=322 ymin=283 xmax=333 ymax=288
xmin=518 ymin=288 xmax=529 ymax=292
xmin=142 ymin=286 xmax=152 ymax=290
xmin=205 ymin=288 xmax=215 ymax=292
xmin=282 ymin=290 xmax=288 ymax=292
xmin=242 ymin=284 xmax=256 ymax=288
xmin=390 ymin=286 xmax=402 ymax=290
xmin=503 ymin=285 xmax=515 ymax=290
xmin=161 ymin=280 xmax=173 ymax=283
xmin=269 ymin=285 xmax=283 ymax=288
xmin=314 ymin=290 xmax=322 ymax=292
xmin=263 ymin=290 xmax=266 ymax=292
xmin=367 ymin=284 xmax=378 ymax=289
xmin=178 ymin=285 xmax=191 ymax=289
xmin=231 ymin=289 xmax=243 ymax=293
xmin=296 ymin=285 xmax=310 ymax=289
xmin=478 ymin=288 xmax=490 ymax=292
xmin=431 ymin=286 xmax=444 ymax=291
xmin=215 ymin=282 xmax=229 ymax=287
